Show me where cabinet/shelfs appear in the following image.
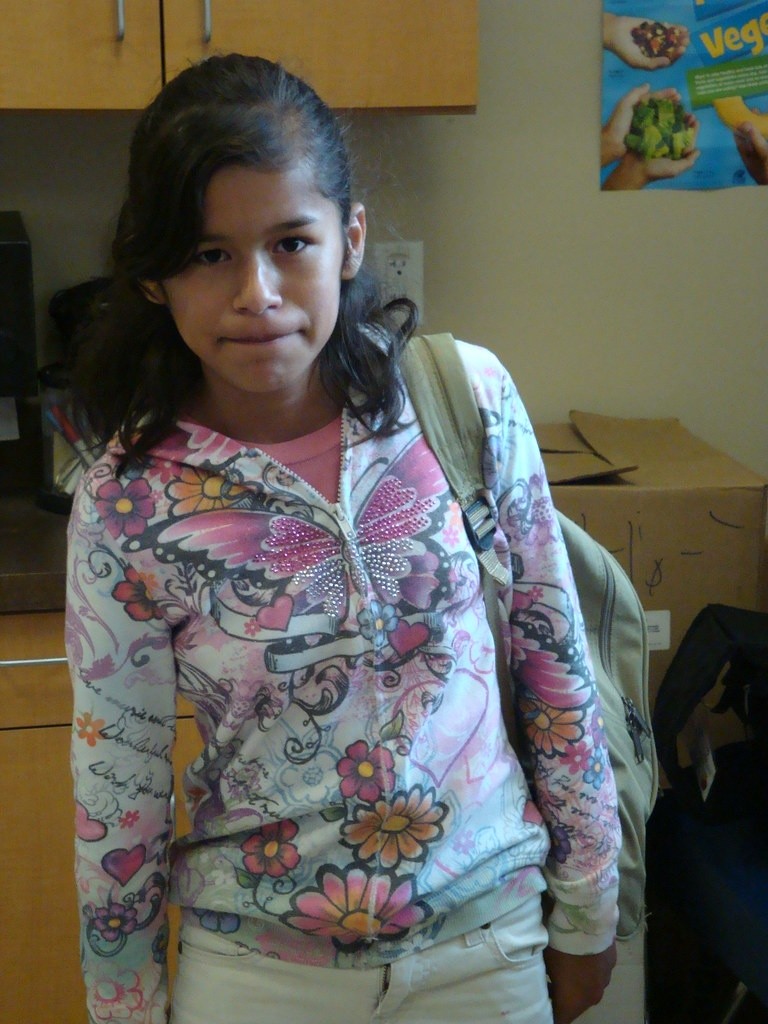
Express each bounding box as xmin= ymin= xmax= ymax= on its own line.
xmin=0 ymin=607 xmax=206 ymax=1024
xmin=0 ymin=0 xmax=480 ymax=116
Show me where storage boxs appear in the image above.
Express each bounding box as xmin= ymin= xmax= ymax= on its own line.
xmin=532 ymin=410 xmax=768 ymax=769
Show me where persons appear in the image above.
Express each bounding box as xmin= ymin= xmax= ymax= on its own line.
xmin=601 ymin=12 xmax=768 ymax=190
xmin=61 ymin=56 xmax=620 ymax=1024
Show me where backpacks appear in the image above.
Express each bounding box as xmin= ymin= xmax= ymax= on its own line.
xmin=643 ymin=603 xmax=768 ymax=1024
xmin=398 ymin=331 xmax=659 ymax=943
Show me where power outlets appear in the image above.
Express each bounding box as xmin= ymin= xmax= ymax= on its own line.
xmin=375 ymin=240 xmax=424 ymax=327
